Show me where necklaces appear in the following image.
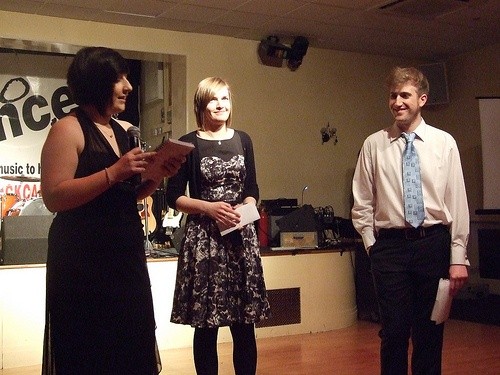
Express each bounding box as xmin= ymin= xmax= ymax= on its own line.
xmin=205 ymin=130 xmax=229 ymax=146
xmin=97 ymin=125 xmax=113 ymax=139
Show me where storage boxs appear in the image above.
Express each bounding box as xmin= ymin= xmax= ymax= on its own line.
xmin=279 ymin=232 xmax=318 ymax=249
xmin=454 ymin=283 xmax=489 ymax=300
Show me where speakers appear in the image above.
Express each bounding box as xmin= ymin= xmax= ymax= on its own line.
xmin=2 ymin=216 xmax=54 ymax=265
xmin=415 ymin=62 xmax=450 ymax=108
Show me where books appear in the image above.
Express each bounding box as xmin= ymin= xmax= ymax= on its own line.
xmin=141 ymin=138 xmax=194 ymax=183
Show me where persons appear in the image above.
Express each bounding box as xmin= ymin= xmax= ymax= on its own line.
xmin=352 ymin=68 xmax=471 ymax=375
xmin=167 ymin=76 xmax=273 ymax=375
xmin=40 ymin=46 xmax=187 ymax=375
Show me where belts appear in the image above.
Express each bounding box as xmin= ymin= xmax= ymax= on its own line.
xmin=378 ymin=224 xmax=447 ymax=241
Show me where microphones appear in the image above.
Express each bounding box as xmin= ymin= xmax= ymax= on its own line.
xmin=127 ymin=127 xmax=142 ymax=185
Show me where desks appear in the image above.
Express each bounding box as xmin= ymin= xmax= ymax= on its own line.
xmin=0 ymin=247 xmax=359 ymax=369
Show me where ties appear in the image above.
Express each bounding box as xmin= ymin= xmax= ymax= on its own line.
xmin=401 ymin=132 xmax=426 ymax=229
